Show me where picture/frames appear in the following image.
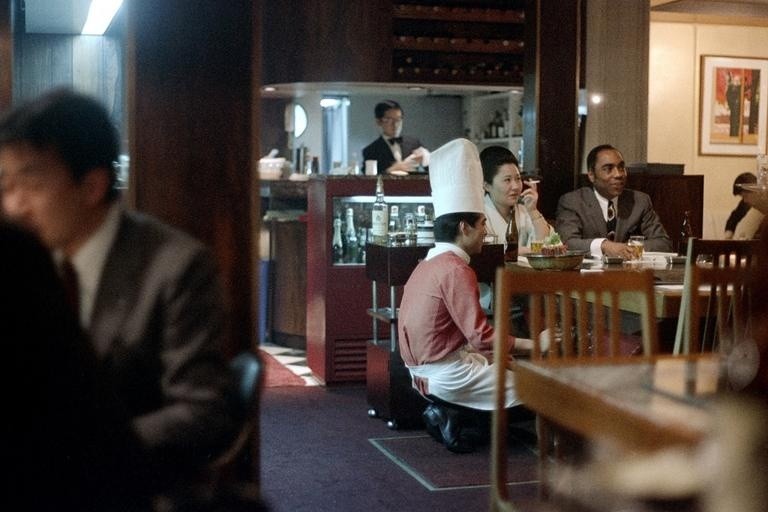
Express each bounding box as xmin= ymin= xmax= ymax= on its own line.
xmin=699 ymin=54 xmax=768 ymax=158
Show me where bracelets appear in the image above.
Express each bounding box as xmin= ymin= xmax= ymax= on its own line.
xmin=531 ymin=213 xmax=542 ymax=221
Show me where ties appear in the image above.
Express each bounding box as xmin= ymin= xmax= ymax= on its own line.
xmin=58 ymin=254 xmax=91 ymax=340
xmin=604 ymin=200 xmax=619 ymax=243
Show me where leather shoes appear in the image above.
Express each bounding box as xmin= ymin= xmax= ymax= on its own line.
xmin=422 ymin=402 xmax=474 ymax=456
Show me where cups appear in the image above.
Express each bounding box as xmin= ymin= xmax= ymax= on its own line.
xmin=364 ymin=159 xmax=378 ymax=176
xmin=626 ymin=235 xmax=645 ymax=260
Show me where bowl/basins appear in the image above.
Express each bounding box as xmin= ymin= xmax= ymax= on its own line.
xmin=256 ymin=158 xmax=286 ymax=180
xmin=520 ymin=250 xmax=592 ymax=270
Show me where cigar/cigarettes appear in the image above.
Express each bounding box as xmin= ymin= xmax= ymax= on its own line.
xmin=529 ymin=180 xmax=540 ymax=184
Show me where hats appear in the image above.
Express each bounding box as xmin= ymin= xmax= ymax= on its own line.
xmin=426 ymin=136 xmax=489 ymax=220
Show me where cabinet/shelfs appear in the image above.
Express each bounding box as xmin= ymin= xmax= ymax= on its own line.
xmin=374 ymin=3 xmax=527 ymax=87
xmin=305 ymin=173 xmax=433 ymax=386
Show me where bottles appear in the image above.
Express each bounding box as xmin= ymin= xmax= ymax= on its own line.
xmin=488 ymin=108 xmax=509 ymax=138
xmin=332 ymin=173 xmax=430 ymax=264
xmin=505 ymin=205 xmax=519 ymax=262
xmin=677 ymin=215 xmax=693 ymax=257
xmin=295 ymin=145 xmax=320 ymax=176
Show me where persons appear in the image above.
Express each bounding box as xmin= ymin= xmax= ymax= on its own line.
xmin=1 ymin=221 xmax=161 ymax=511
xmin=724 ymin=172 xmax=756 ymax=238
xmin=397 ymin=213 xmax=561 ymax=455
xmin=555 ymin=144 xmax=673 ymax=260
xmin=479 ymin=146 xmax=562 ymax=330
xmin=1 ymin=88 xmax=248 ymax=512
xmin=360 ymin=99 xmax=431 ymax=174
xmin=725 ymin=74 xmax=741 ymax=137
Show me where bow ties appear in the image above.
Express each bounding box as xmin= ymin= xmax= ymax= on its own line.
xmin=387 ymin=135 xmax=404 ymax=146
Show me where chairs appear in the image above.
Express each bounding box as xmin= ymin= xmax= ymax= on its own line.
xmin=158 ymin=351 xmax=263 ymax=512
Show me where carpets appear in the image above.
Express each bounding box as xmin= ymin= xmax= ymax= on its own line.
xmin=369 ymin=435 xmax=558 ymax=491
